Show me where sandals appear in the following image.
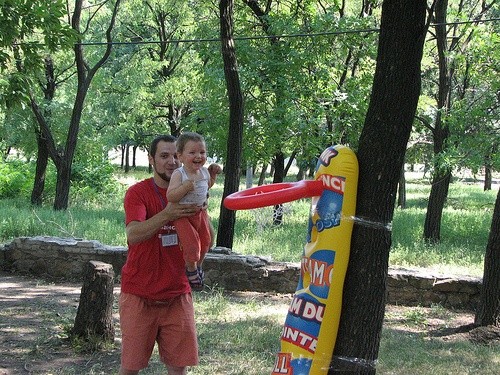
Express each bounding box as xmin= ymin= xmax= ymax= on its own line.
xmin=185 ymin=267 xmax=205 ymax=292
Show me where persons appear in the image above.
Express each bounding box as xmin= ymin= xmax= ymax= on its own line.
xmin=118 ymin=135 xmax=209 ymax=375
xmin=166 ymin=132 xmax=222 ymax=292
